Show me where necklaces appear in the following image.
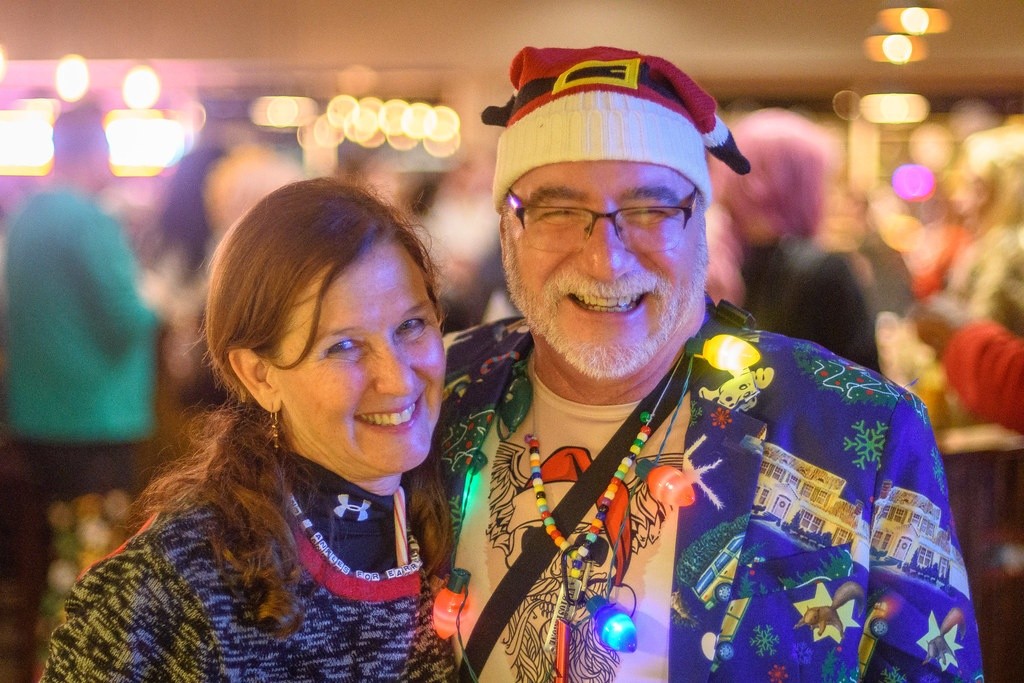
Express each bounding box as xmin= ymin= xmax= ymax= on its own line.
xmin=288 ymin=493 xmax=423 ymax=581
xmin=529 ymin=354 xmax=685 ymax=581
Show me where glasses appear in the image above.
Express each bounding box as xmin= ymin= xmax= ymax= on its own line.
xmin=508 ymin=187 xmax=699 ymax=253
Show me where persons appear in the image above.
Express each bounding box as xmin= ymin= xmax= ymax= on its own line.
xmin=408 ymin=45 xmax=984 ymax=683
xmin=38 ymin=177 xmax=446 ymax=683
xmin=7 ymin=111 xmax=162 ymax=501
xmin=918 ymin=296 xmax=1024 ymax=434
xmin=707 ymin=106 xmax=881 ymax=375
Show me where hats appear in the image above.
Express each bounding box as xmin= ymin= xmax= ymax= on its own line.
xmin=482 ymin=47 xmax=751 ymax=212
